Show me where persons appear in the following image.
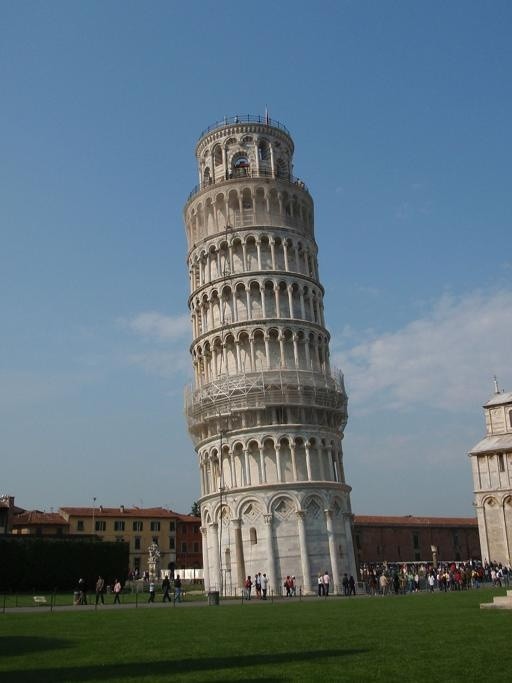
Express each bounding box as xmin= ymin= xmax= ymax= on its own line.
xmin=74 ymin=561 xmax=511 ymax=606
